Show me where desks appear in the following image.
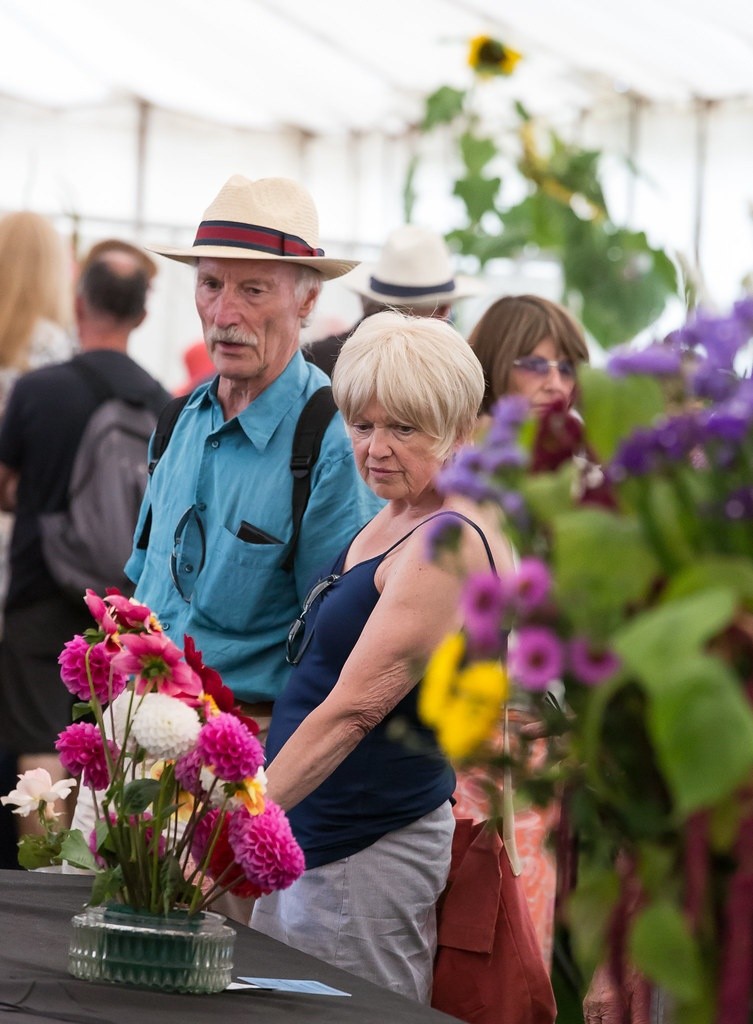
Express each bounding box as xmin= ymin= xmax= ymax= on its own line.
xmin=0 ymin=870 xmax=464 ymax=1024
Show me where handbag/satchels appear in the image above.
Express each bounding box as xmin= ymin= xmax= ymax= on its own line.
xmin=429 ymin=815 xmax=558 ymax=1024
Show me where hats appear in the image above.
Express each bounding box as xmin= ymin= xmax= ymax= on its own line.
xmin=345 ymin=216 xmax=476 ymax=305
xmin=141 ymin=171 xmax=360 ymax=281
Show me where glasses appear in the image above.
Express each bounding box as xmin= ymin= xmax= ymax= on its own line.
xmin=168 ymin=503 xmax=205 ymax=605
xmin=283 ymin=574 xmax=341 ymax=668
xmin=510 ymin=356 xmax=575 ymax=376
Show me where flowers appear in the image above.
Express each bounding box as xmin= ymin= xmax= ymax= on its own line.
xmin=1 ymin=584 xmax=307 ymax=919
xmin=408 ymin=36 xmax=753 ymax=1024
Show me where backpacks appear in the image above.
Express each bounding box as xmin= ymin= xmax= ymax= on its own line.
xmin=30 ymin=356 xmax=159 ymax=600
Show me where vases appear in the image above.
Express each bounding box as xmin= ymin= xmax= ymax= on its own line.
xmin=67 ymin=910 xmax=235 ymax=995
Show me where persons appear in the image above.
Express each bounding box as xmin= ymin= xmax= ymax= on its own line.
xmin=0 ymin=171 xmax=752 ymax=1024
